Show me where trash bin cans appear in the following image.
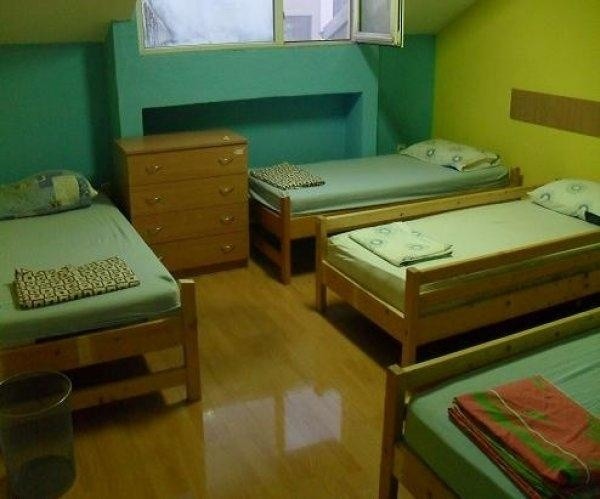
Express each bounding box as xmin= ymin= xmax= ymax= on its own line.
xmin=0 ymin=369 xmax=75 ymax=499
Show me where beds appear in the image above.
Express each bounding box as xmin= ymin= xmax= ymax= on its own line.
xmin=314 ymin=183 xmax=599 ymax=368
xmin=380 ymin=307 xmax=600 ymax=499
xmin=0 ymin=186 xmax=202 ymax=428
xmin=249 ymin=154 xmax=521 ymax=285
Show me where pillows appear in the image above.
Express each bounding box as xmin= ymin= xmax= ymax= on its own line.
xmin=399 ymin=138 xmax=501 ymax=172
xmin=0 ymin=170 xmax=98 ymax=220
xmin=527 ymin=177 xmax=600 ymax=225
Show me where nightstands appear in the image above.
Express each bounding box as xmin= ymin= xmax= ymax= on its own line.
xmin=114 ymin=128 xmax=249 ymax=279
